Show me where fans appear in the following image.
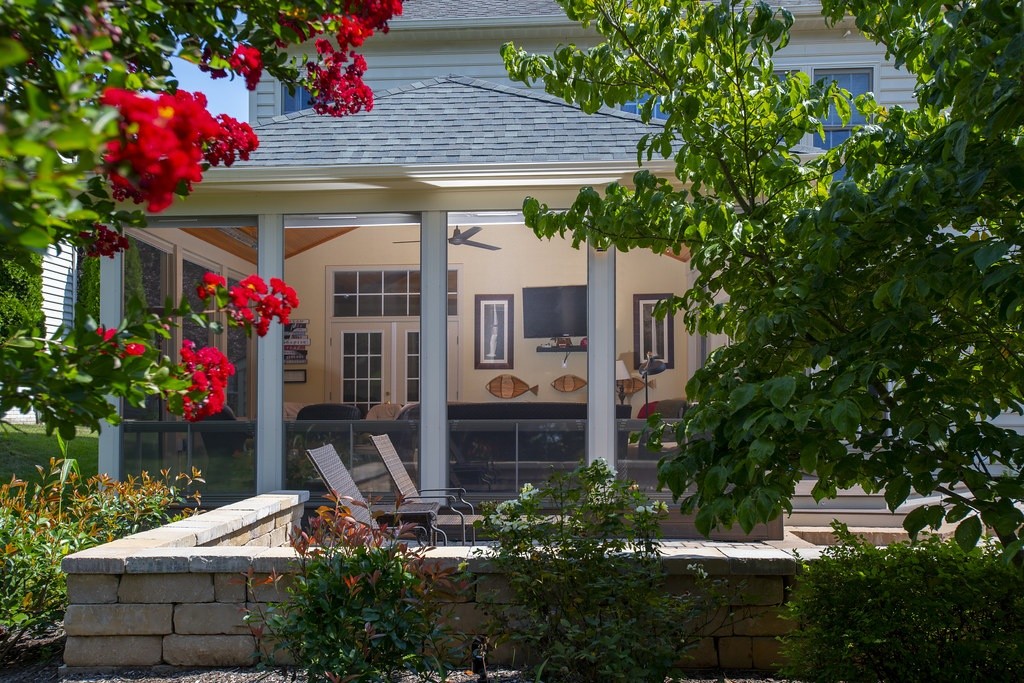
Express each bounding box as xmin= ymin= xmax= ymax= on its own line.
xmin=393 ymin=222 xmax=500 ymax=257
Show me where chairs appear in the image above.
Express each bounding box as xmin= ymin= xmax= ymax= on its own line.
xmin=638 ymin=399 xmax=692 ymax=446
xmin=306 ymin=444 xmax=467 ymax=545
xmin=367 ymin=433 xmax=485 ymax=547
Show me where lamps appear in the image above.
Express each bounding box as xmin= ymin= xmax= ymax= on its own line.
xmin=637 ymin=352 xmax=667 ymax=448
xmin=615 ymin=361 xmax=631 ymax=405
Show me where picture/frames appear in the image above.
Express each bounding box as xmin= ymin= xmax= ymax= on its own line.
xmin=474 ymin=292 xmax=515 ymax=369
xmin=632 ymin=293 xmax=675 ymax=370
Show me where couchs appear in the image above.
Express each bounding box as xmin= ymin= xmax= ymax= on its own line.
xmin=200 ymin=398 xmax=633 ymax=492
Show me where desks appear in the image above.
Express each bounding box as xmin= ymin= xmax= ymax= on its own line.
xmin=372 ymin=502 xmax=441 ymax=544
